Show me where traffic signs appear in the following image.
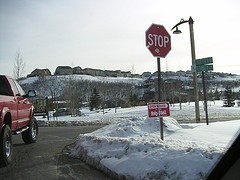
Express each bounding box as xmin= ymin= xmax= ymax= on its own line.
xmin=193 ymin=57 xmax=213 ymax=65
xmin=191 ymin=64 xmax=214 ymax=73
xmin=147 ymin=101 xmax=170 ymax=118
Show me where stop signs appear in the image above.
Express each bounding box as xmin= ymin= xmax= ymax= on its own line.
xmin=146 ymin=23 xmax=172 ymax=58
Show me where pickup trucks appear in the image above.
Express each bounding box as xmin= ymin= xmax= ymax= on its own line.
xmin=0 ymin=73 xmax=39 ymax=168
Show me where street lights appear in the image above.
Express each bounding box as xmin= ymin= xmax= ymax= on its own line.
xmin=169 ymin=17 xmax=201 ymax=122
